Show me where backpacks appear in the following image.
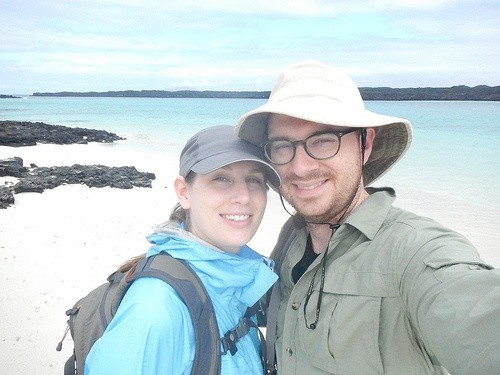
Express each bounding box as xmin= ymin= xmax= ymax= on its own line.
xmin=57 ymin=249 xmax=265 ymax=374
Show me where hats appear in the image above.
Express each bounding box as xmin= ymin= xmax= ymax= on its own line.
xmin=235 ymin=60 xmax=413 ymax=188
xmin=178 ymin=125 xmax=282 ymax=189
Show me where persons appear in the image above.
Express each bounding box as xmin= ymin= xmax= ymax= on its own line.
xmin=233 ymin=59 xmax=500 ymax=375
xmin=84 ymin=125 xmax=281 ymax=375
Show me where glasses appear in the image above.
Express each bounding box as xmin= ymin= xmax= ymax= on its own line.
xmin=258 ymin=127 xmax=365 ymax=166
